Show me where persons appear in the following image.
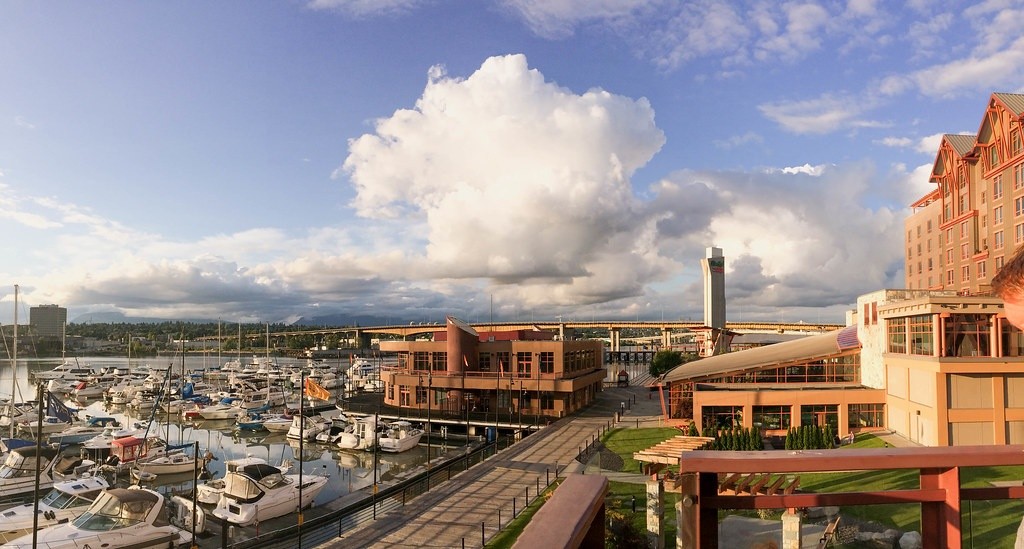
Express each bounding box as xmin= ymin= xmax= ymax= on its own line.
xmin=990 ymin=244 xmax=1024 ymax=549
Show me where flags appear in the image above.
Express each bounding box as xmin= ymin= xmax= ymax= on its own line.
xmin=463 ymin=355 xmax=469 ymax=367
xmin=350 ymin=353 xmax=353 ymax=364
xmin=500 ymin=359 xmax=504 ymax=378
xmin=305 ymin=378 xmax=331 ymax=402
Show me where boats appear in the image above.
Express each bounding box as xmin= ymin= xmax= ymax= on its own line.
xmin=30 ymin=315 xmax=425 ymax=453
xmin=0 ymin=396 xmax=195 ymax=474
xmin=0 ymin=484 xmax=206 ymax=549
xmin=196 ymin=456 xmax=330 ymax=527
xmin=0 ymin=472 xmax=112 ymax=545
xmin=136 ymin=452 xmax=203 ymax=475
xmin=0 ymin=443 xmax=92 ymax=511
